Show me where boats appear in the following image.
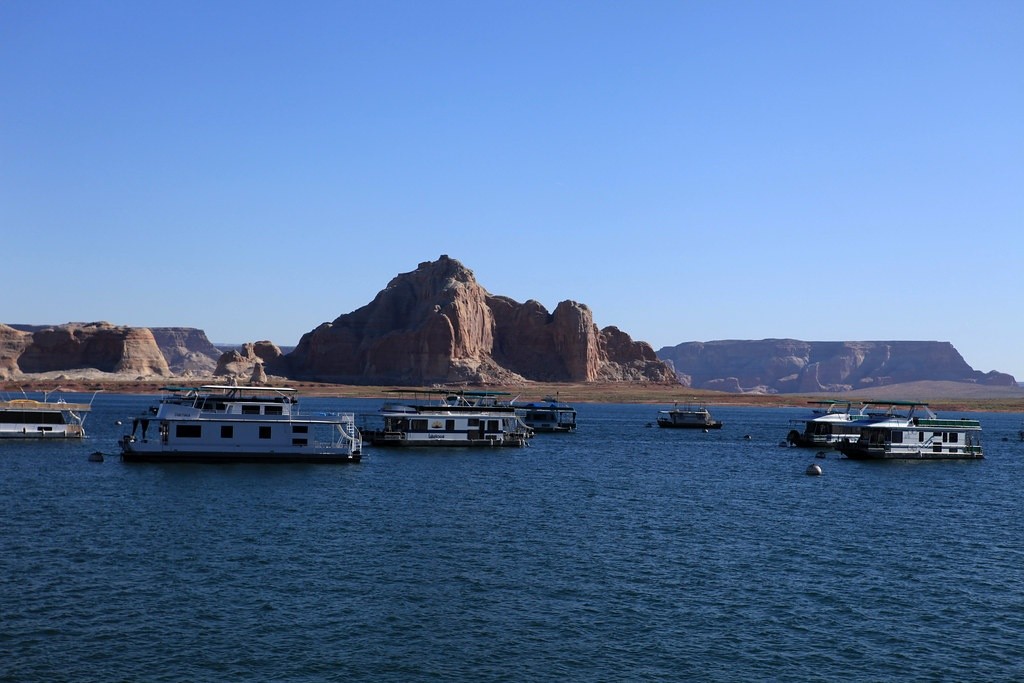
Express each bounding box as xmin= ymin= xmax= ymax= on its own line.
xmin=830 ymin=400 xmax=985 ymax=461
xmin=0 ymin=399 xmax=92 ymax=439
xmin=657 ymin=402 xmax=723 ymax=430
xmin=118 ymin=384 xmax=363 ymax=463
xmin=357 ymin=387 xmax=534 ymax=449
xmin=786 ymin=401 xmax=906 ymax=450
xmin=465 ymin=391 xmax=577 ymax=433
